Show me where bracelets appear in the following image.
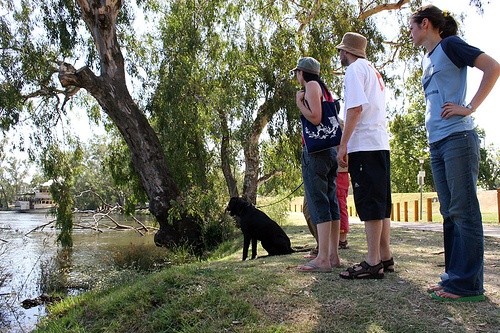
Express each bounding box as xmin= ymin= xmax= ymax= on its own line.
xmin=466 ymin=103 xmax=476 ymax=112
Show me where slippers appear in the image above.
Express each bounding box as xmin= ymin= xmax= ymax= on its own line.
xmin=427 ymin=285 xmax=443 ymax=294
xmin=297 ymin=263 xmax=333 ymax=273
xmin=431 ymin=288 xmax=486 ymax=303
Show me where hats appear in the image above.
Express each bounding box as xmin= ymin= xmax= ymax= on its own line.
xmin=291 ymin=56 xmax=321 ymax=75
xmin=335 ymin=32 xmax=367 ymax=58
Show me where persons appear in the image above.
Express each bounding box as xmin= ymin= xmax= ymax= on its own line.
xmin=292 ymin=57 xmax=349 ymax=271
xmin=336 ymin=32 xmax=395 ymax=279
xmin=410 ymin=5 xmax=500 ymax=302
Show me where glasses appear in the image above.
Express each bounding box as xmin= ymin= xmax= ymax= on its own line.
xmin=338 ymin=49 xmax=342 ymax=53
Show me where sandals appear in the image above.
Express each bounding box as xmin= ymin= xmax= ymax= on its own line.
xmin=339 ymin=261 xmax=385 ymax=280
xmin=338 ymin=239 xmax=349 ymax=249
xmin=353 ymin=257 xmax=395 ymax=272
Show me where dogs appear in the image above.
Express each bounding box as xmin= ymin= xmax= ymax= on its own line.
xmin=226 ymin=197 xmax=295 ymax=262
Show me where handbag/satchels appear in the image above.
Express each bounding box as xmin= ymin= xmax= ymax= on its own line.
xmin=300 ymin=81 xmax=342 ymax=155
